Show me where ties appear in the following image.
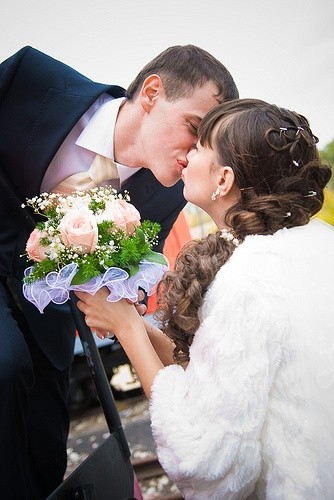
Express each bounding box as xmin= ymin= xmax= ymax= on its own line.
xmin=43 ymin=155 xmax=118 ymax=209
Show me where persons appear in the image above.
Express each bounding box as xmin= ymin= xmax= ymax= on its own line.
xmin=70 ymin=97 xmax=334 ymax=500
xmin=0 ymin=43 xmax=242 ymax=500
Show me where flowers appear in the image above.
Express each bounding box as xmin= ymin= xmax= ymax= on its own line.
xmin=19 ymin=185 xmax=168 ymax=284
